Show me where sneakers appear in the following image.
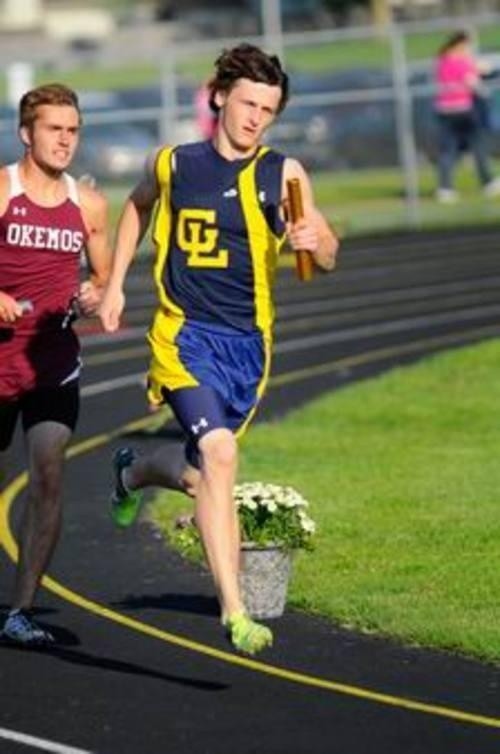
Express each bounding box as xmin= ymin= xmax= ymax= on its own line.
xmin=0 ymin=608 xmax=56 ymax=649
xmin=220 ymin=607 xmax=274 ymax=654
xmin=107 ymin=444 xmax=144 ymax=529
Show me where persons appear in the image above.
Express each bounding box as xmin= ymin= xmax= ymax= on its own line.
xmin=431 ymin=28 xmax=500 ymax=203
xmin=195 ymin=77 xmax=215 ymax=138
xmin=99 ymin=41 xmax=341 ymax=657
xmin=1 ymin=81 xmax=113 ymax=647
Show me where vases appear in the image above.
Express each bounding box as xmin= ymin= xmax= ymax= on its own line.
xmin=237 ymin=541 xmax=289 ymax=621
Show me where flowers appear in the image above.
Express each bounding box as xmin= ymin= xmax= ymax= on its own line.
xmin=230 ymin=481 xmax=320 ymax=557
